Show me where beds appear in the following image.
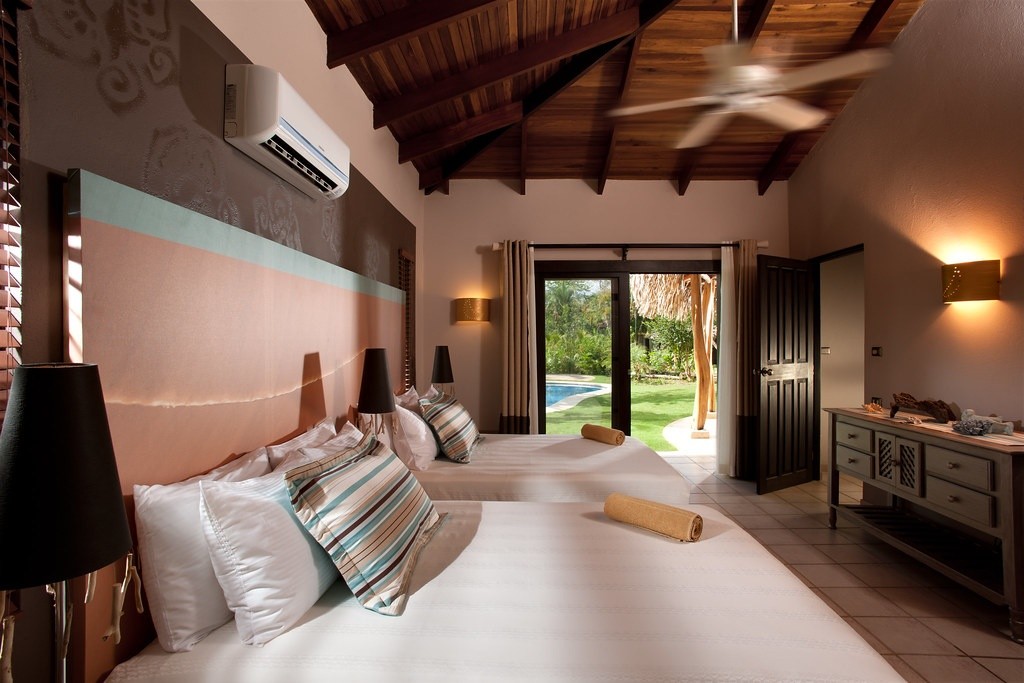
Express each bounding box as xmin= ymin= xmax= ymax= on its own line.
xmin=62 ymin=168 xmax=906 ymax=682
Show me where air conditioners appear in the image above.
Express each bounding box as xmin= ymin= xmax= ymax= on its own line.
xmin=224 ymin=64 xmax=351 ymax=200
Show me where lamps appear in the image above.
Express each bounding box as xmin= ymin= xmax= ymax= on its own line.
xmin=942 ymin=260 xmax=1001 ymax=305
xmin=358 ymin=349 xmax=396 ymax=436
xmin=431 ymin=346 xmax=454 ymax=392
xmin=0 ymin=362 xmax=132 ymax=683
xmin=454 ymin=298 xmax=490 ymax=324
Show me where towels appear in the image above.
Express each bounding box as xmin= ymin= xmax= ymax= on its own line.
xmin=581 ymin=424 xmax=625 ymax=446
xmin=603 ymin=492 xmax=703 ymax=544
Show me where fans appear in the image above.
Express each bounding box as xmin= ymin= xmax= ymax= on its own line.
xmin=607 ymin=1 xmax=891 ymax=149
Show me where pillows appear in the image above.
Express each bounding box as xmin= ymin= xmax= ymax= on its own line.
xmin=348 ymin=405 xmax=396 ymax=452
xmin=391 ymin=403 xmax=441 ymax=471
xmin=393 ymin=385 xmax=420 ymax=406
xmin=133 ymin=447 xmax=272 ymax=653
xmin=266 ymin=416 xmax=337 ymax=471
xmin=283 ymin=427 xmax=450 ymax=616
xmin=418 ymin=391 xmax=485 ymax=463
xmin=298 ymin=420 xmax=364 ymax=461
xmin=198 ymin=448 xmax=341 ymax=647
xmin=405 ymin=384 xmax=438 ymax=411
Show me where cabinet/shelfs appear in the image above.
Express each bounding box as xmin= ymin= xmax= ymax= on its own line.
xmin=822 ymin=408 xmax=1024 ymax=641
